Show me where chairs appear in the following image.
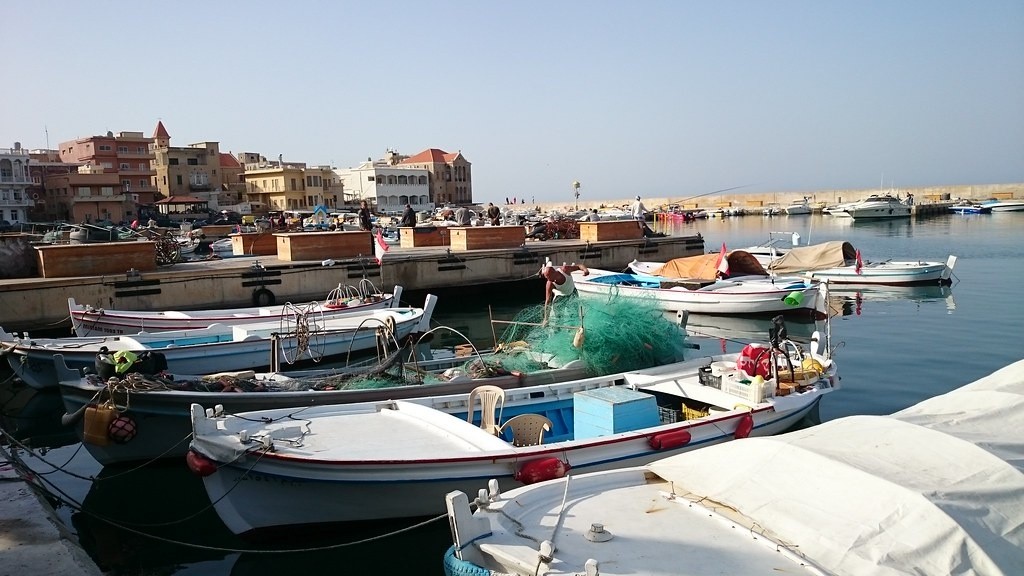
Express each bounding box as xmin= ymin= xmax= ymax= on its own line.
xmin=467 ymin=385 xmax=505 ymax=435
xmin=494 ymin=413 xmax=553 ymax=447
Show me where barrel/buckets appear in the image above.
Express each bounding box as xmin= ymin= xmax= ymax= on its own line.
xmin=710 ymin=361 xmax=738 ymax=378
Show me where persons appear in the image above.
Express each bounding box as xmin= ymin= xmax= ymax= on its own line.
xmin=358 ymin=200 xmax=375 ymax=256
xmin=542 ymin=265 xmax=589 ymax=334
xmin=631 ymin=196 xmax=650 ymax=220
xmin=268 ymin=214 xmax=285 ymax=227
xmin=476 ymin=213 xmax=485 ymax=226
xmin=907 ymin=192 xmax=914 ymax=200
xmin=587 ymin=209 xmax=600 ymax=221
xmin=447 ymin=207 xmax=471 ymax=225
xmin=488 ymin=203 xmax=500 ymax=226
xmin=396 ymin=204 xmax=416 ymax=240
xmin=131 ymin=217 xmax=140 ymax=231
xmin=148 ymin=217 xmax=156 ymax=230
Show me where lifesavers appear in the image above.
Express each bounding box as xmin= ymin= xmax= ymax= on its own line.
xmin=252 ymin=288 xmax=276 ymax=308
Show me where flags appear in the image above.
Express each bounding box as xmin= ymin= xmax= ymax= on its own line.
xmin=713 ymin=242 xmax=730 ymax=276
xmin=854 ymin=249 xmax=862 ymax=274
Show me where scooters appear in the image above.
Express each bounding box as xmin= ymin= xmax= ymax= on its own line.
xmin=515 ymin=214 xmax=550 ymax=242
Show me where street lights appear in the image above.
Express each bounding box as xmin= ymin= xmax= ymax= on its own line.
xmin=573 ymin=180 xmax=580 ymax=211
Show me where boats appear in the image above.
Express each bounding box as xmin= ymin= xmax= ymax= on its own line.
xmin=627 ymin=259 xmax=815 ymax=286
xmin=169 ymin=238 xmax=201 ymax=255
xmin=762 ymin=204 xmax=783 ymax=215
xmin=822 ymin=200 xmax=864 ymax=217
xmin=68 ymin=285 xmax=403 ymax=346
xmin=0 ymin=293 xmax=438 ymax=394
xmin=785 ymin=198 xmax=812 ymax=214
xmin=947 ymin=198 xmax=984 ymax=214
xmin=54 ymin=309 xmax=699 ymax=469
xmin=843 ymin=175 xmax=912 ymax=220
xmin=445 ymin=356 xmax=1024 ymax=576
xmin=708 ymin=231 xmax=958 ymax=286
xmin=186 ymin=313 xmax=840 ymax=536
xmin=206 ymin=238 xmax=233 ymax=251
xmin=543 ymin=257 xmax=829 ymax=318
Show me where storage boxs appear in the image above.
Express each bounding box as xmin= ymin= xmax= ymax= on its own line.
xmin=699 ymin=367 xmax=776 ymax=403
xmin=657 ymin=401 xmax=709 ymax=424
